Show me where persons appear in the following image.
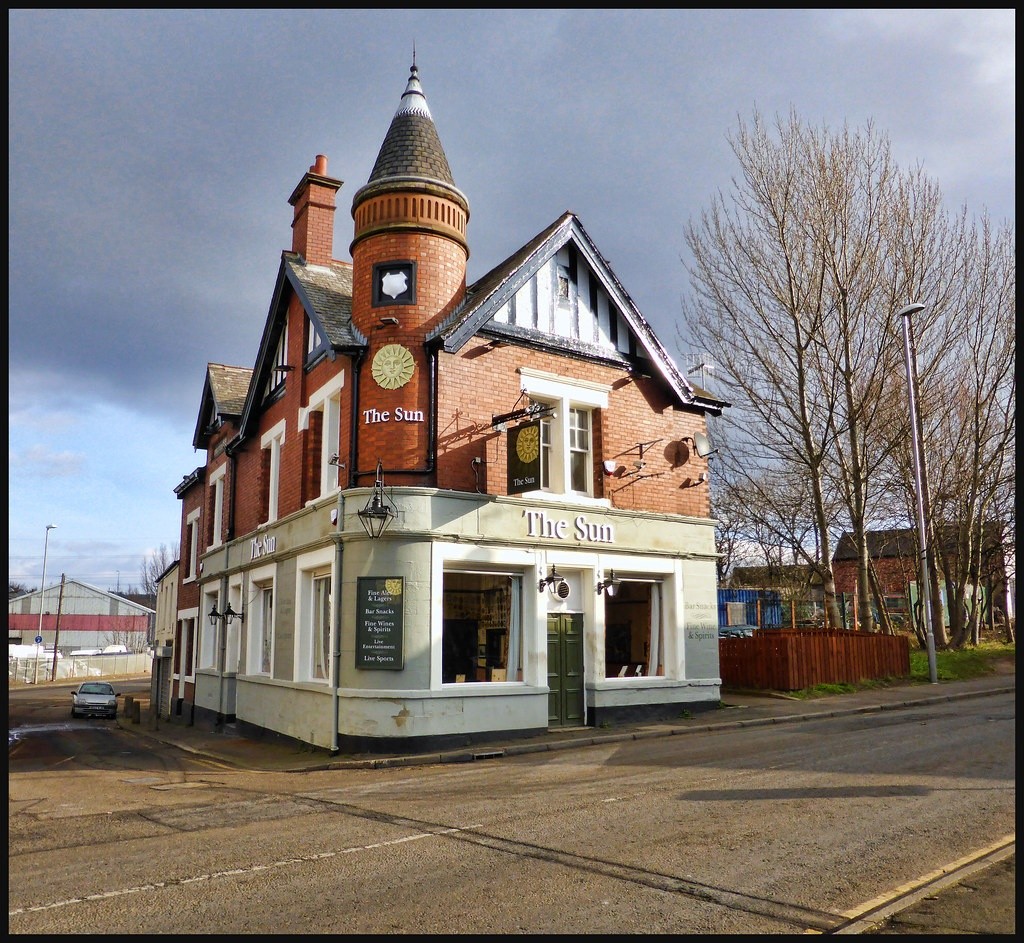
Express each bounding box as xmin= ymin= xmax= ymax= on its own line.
xmin=871 ymin=607 xmax=879 ymax=625
xmin=993 ymin=607 xmax=1005 ymax=623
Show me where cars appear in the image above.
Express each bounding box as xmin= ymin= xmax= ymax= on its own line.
xmin=68 ymin=681 xmax=121 ymax=717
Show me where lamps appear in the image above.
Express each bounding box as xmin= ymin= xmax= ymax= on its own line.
xmin=358 ymin=461 xmax=398 ymax=539
xmin=273 ymin=365 xmax=295 ymax=372
xmin=484 ymin=340 xmax=511 ymax=350
xmin=208 ymin=604 xmax=225 ymax=625
xmin=629 ymin=373 xmax=651 ymax=381
xmin=223 ymin=602 xmax=244 ymax=624
xmin=376 ymin=317 xmax=399 ymax=329
xmin=540 ymin=563 xmax=564 ymax=593
xmin=598 ymin=568 xmax=621 ymax=597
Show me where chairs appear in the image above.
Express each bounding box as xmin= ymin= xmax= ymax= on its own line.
xmin=101 ymin=688 xmax=106 ymax=693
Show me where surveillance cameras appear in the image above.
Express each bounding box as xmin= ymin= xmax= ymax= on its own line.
xmin=328 ymin=453 xmax=339 ymax=465
xmin=698 ymin=473 xmax=706 ymax=482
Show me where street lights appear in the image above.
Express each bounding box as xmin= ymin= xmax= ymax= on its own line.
xmin=34 ymin=524 xmax=58 ymax=685
xmin=898 ymin=303 xmax=938 ymax=683
